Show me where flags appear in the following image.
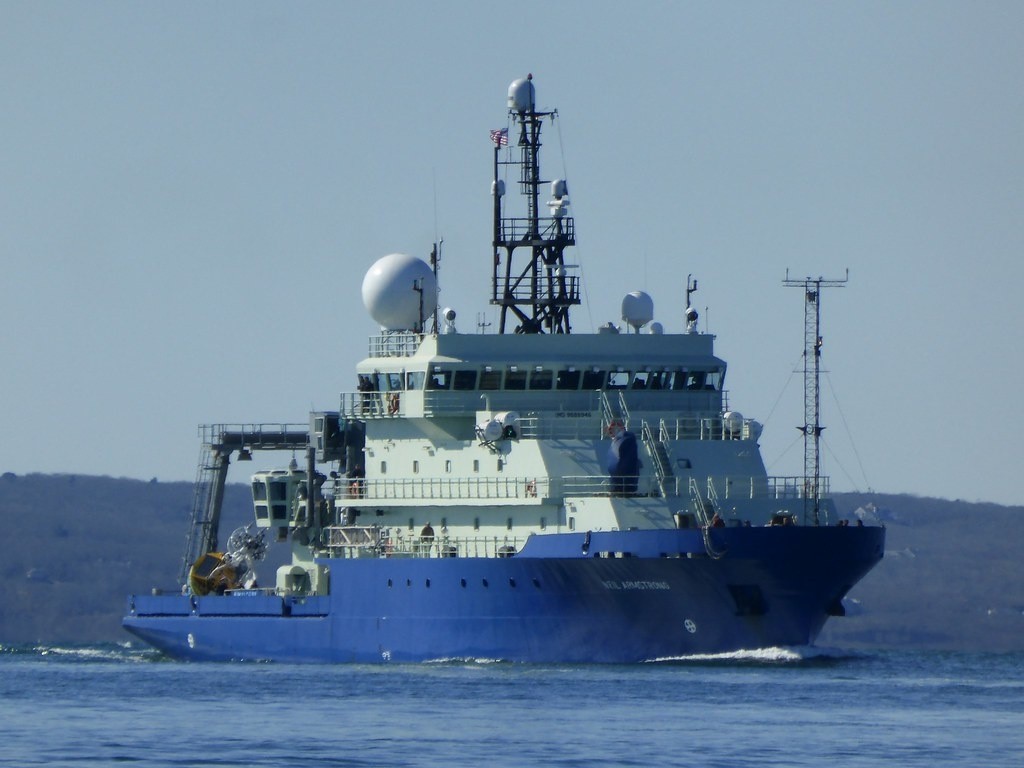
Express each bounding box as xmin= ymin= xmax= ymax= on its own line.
xmin=489 ymin=128 xmax=508 ymax=146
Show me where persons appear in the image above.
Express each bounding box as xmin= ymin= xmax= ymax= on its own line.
xmin=359 ymin=376 xmax=374 ymax=413
xmin=421 ymin=521 xmax=434 ymax=557
xmin=216 ymin=577 xmax=230 ymax=596
xmin=350 ymin=463 xmax=363 ymax=498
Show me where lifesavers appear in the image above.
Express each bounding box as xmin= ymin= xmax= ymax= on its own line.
xmin=606 ymin=422 xmax=625 ymax=439
xmin=351 ymin=483 xmax=359 ymax=498
xmin=385 ymin=539 xmax=393 ymax=557
xmin=527 ymin=481 xmax=536 ymax=497
xmin=388 ymin=395 xmax=396 ymax=413
xmin=803 ymin=480 xmax=810 ymax=495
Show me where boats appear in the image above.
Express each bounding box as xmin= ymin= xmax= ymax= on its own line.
xmin=121 ymin=74 xmax=885 ymax=666
